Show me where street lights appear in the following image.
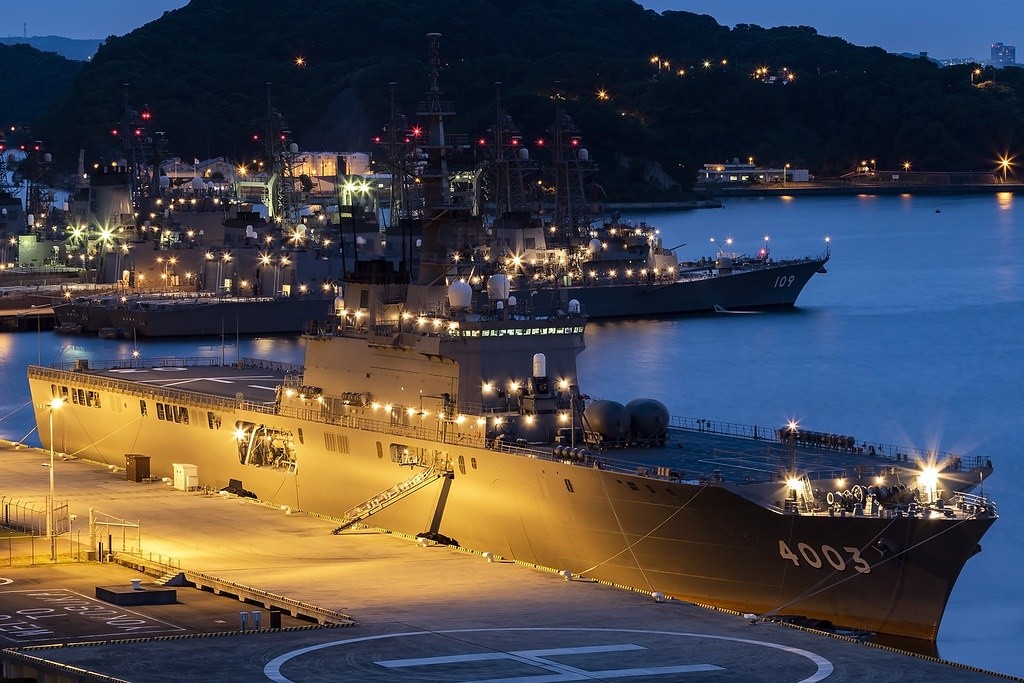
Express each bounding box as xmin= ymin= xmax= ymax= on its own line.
xmin=783 ymin=164 xmax=791 ymax=187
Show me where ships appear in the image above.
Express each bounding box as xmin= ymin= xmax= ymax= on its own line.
xmin=26 ymin=31 xmax=1001 ymax=660
xmin=53 ymin=77 xmax=831 ymax=344
